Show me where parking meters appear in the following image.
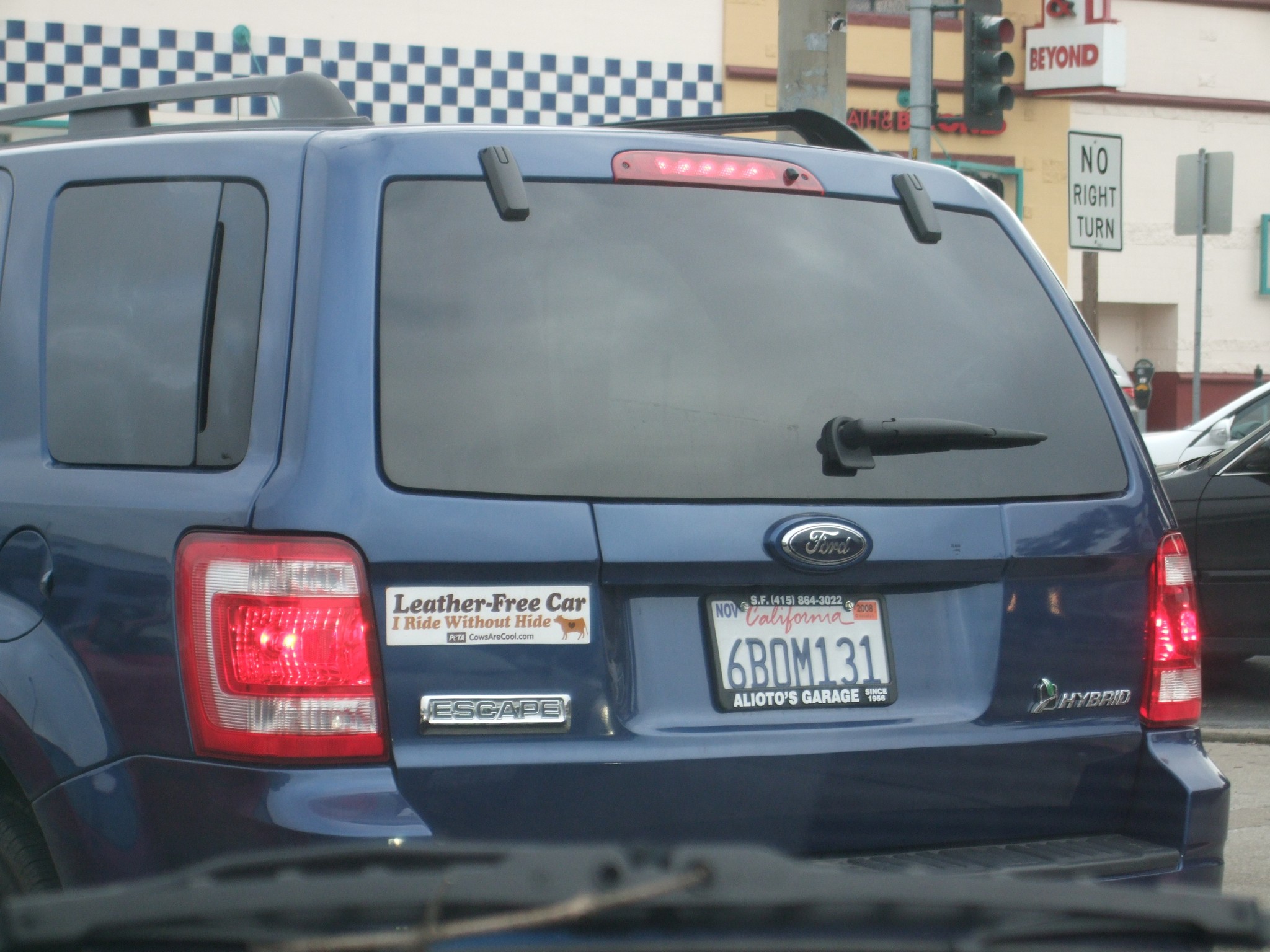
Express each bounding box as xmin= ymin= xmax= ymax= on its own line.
xmin=1134 ymin=359 xmax=1155 ymax=433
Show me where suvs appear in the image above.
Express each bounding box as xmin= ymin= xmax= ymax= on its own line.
xmin=1 ymin=74 xmax=1236 ymax=952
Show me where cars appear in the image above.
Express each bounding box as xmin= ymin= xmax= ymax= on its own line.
xmin=1138 ymin=379 xmax=1270 ymax=658
xmin=1101 ymin=349 xmax=1148 ymax=434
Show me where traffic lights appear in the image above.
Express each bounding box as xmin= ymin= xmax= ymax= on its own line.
xmin=962 ymin=0 xmax=1015 ymax=128
